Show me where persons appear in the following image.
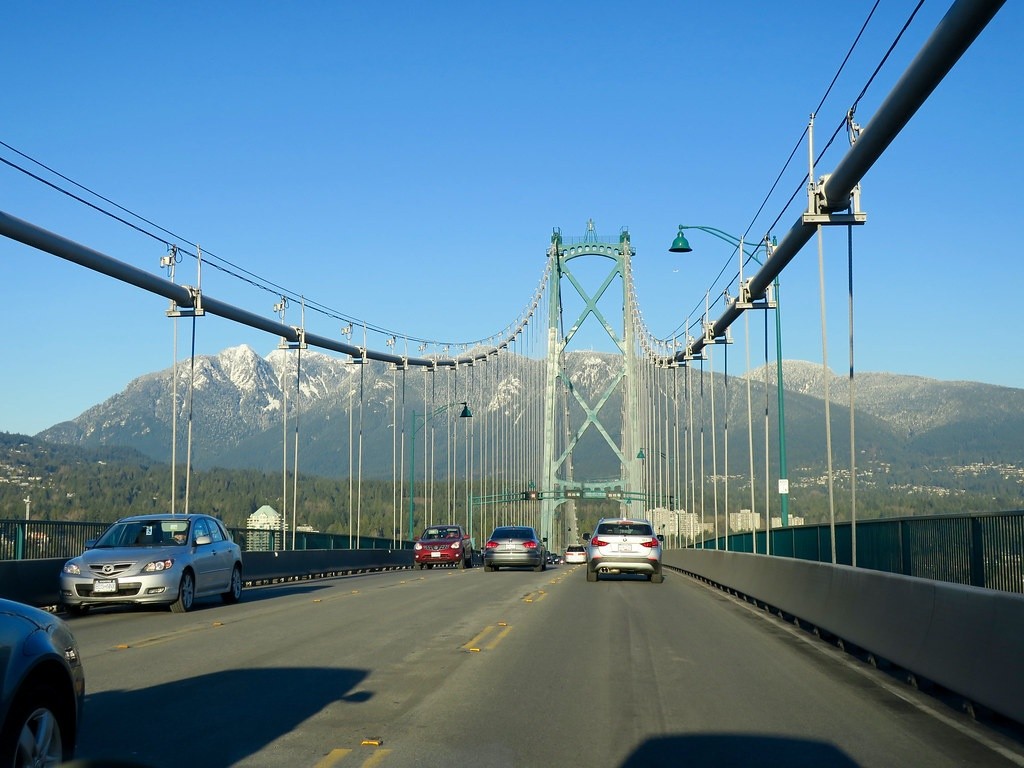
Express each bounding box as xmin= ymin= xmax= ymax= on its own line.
xmin=173 ymin=531 xmax=187 ymax=545
xmin=448 ymin=532 xmax=456 ymax=538
xmin=433 ymin=534 xmax=438 ymax=539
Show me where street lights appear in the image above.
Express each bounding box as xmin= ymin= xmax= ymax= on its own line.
xmin=669 ymin=224 xmax=789 ymax=529
xmin=409 ymin=401 xmax=473 ymax=539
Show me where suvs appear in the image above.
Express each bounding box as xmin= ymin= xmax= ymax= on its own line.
xmin=413 ymin=525 xmax=474 ymax=570
xmin=587 ymin=517 xmax=665 ymax=584
xmin=565 ymin=545 xmax=587 ymax=564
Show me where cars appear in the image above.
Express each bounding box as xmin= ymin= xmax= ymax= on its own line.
xmin=0 ymin=598 xmax=86 ymax=768
xmin=481 ymin=527 xmax=550 ymax=572
xmin=62 ymin=514 xmax=245 ymax=614
xmin=546 ymin=552 xmax=564 ymax=565
